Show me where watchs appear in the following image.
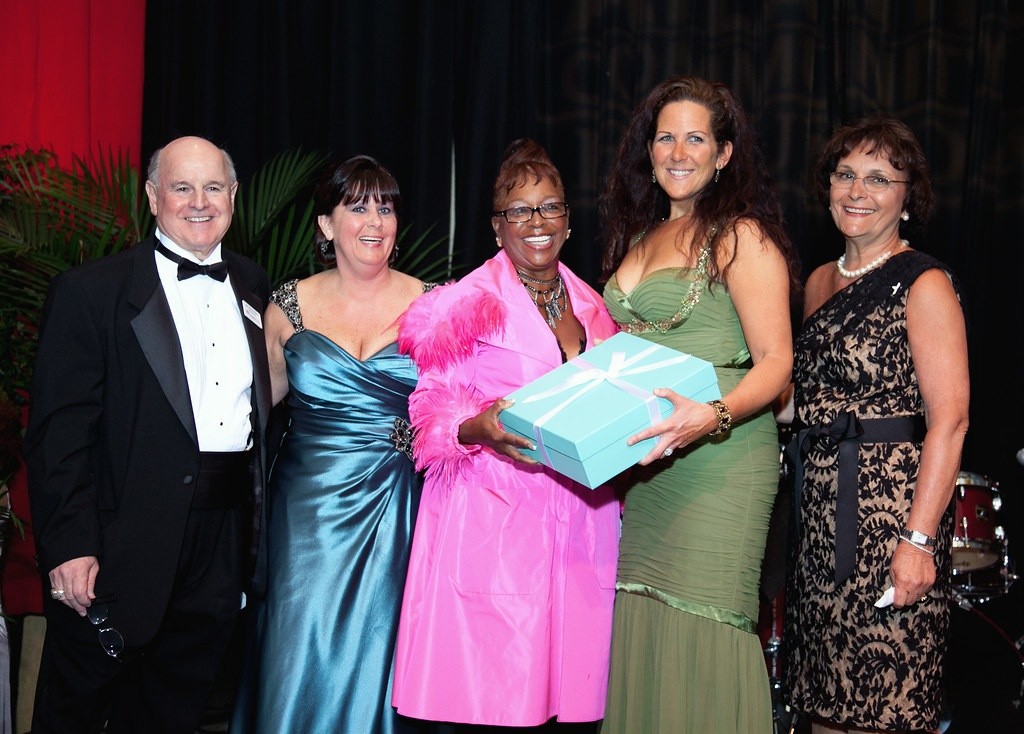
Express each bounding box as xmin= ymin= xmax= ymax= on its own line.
xmin=902 ymin=528 xmax=937 ymax=546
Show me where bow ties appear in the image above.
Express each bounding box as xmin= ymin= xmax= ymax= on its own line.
xmin=154 ymin=236 xmax=230 ymax=282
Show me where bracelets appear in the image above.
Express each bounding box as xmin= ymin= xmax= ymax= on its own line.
xmin=900 ymin=535 xmax=938 ymax=556
xmin=706 ymin=399 xmax=734 ymax=444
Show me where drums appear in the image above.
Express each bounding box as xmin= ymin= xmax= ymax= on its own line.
xmin=950 ymin=472 xmax=1010 ymax=576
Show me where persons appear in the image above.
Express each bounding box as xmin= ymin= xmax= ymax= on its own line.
xmin=25 ymin=135 xmax=271 ymax=734
xmin=390 ymin=138 xmax=621 ymax=734
xmin=600 ymin=76 xmax=794 ymax=734
xmin=258 ymin=155 xmax=439 ymax=734
xmin=774 ymin=115 xmax=971 ymax=734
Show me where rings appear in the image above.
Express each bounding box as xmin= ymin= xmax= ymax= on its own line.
xmin=50 ymin=588 xmax=65 ymax=600
xmin=919 ymin=595 xmax=927 ymax=602
xmin=664 ymin=448 xmax=673 ymax=457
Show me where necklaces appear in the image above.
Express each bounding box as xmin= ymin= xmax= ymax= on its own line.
xmin=515 ymin=269 xmax=567 ymax=329
xmin=837 ymin=239 xmax=909 ymax=278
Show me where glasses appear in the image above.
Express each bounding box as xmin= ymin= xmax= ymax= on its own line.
xmin=493 ymin=203 xmax=569 ymax=223
xmin=85 ymin=591 xmax=145 ymax=663
xmin=830 ymin=170 xmax=913 ymax=193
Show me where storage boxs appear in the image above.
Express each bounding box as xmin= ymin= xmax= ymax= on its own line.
xmin=497 ymin=332 xmax=724 ymax=491
